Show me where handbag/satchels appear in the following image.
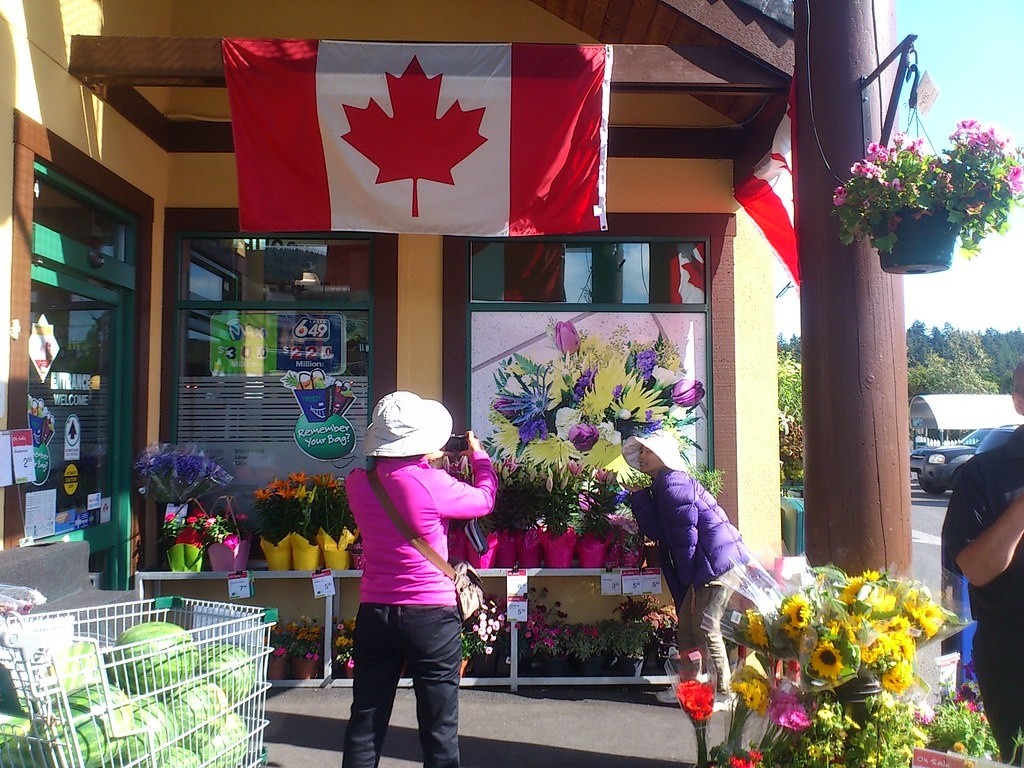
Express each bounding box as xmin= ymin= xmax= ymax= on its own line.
xmin=453 ymin=560 xmax=485 ymax=620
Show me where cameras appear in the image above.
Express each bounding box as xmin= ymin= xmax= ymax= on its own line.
xmin=445 ymin=436 xmax=467 ymax=451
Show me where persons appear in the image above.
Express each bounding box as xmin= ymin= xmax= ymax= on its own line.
xmin=342 ymin=389 xmax=499 ymax=768
xmin=619 ymin=425 xmax=752 ymax=715
xmin=941 ymin=356 xmax=1024 ymax=765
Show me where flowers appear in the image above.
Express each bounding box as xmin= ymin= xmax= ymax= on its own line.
xmin=135 ymin=449 xmax=230 ymax=499
xmin=164 ymin=515 xmax=208 ymax=548
xmin=206 ymin=513 xmax=248 ymax=548
xmin=251 ymin=457 xmax=662 ymax=538
xmin=832 ymin=120 xmax=1024 ymax=253
xmin=264 ymin=587 xmax=679 ymax=661
xmin=661 ymin=556 xmax=1021 ymax=767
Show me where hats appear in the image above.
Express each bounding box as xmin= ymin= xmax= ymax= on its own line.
xmin=362 ymin=391 xmax=453 ymax=457
xmin=621 ymin=430 xmax=688 ymax=472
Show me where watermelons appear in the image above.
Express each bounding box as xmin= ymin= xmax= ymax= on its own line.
xmin=0 ymin=621 xmax=255 ymax=768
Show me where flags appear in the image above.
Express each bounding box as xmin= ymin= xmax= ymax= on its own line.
xmin=669 ymin=243 xmax=708 ymax=303
xmin=324 ymin=228 xmax=567 ymax=437
xmin=729 ymin=68 xmax=804 ymax=285
xmin=218 ymin=32 xmax=613 ymax=240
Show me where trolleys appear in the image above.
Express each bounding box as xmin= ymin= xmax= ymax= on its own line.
xmin=0 ymin=595 xmax=281 ymax=768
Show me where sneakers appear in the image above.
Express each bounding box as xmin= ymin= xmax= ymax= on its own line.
xmin=656 ymin=686 xmax=682 ymax=704
xmin=711 ymin=692 xmax=736 ymax=714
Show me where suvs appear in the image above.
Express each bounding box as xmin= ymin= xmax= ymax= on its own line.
xmin=909 ymin=422 xmax=1023 ymax=495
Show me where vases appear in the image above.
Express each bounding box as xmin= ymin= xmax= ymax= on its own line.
xmin=835 ymin=670 xmax=885 ymax=728
xmin=267 ymin=651 xmax=678 ymax=679
xmin=208 ymin=541 xmax=252 ymax=572
xmin=167 ymin=543 xmax=205 ymax=573
xmin=265 ymin=532 xmax=615 ymax=575
xmin=863 ymin=206 xmax=967 ymax=275
